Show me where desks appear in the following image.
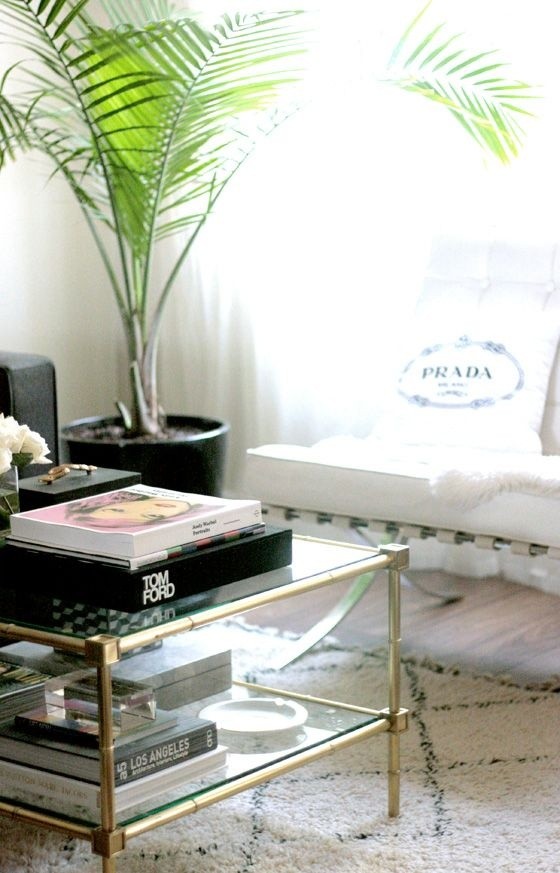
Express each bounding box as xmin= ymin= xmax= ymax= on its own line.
xmin=243 ymin=444 xmax=560 ymax=674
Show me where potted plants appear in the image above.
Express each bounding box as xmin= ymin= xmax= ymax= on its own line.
xmin=0 ymin=0 xmax=310 ymax=620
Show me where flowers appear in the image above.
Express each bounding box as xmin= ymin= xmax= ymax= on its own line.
xmin=0 ymin=411 xmax=53 ymax=474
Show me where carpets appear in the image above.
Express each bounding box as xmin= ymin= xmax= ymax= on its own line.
xmin=0 ymin=616 xmax=560 ymax=873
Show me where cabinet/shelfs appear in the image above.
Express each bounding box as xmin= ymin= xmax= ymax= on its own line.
xmin=0 ymin=530 xmax=411 ymax=873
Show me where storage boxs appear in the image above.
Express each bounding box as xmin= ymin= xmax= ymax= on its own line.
xmin=3 ymin=464 xmax=142 ymax=514
xmin=4 ymin=526 xmax=294 ymax=614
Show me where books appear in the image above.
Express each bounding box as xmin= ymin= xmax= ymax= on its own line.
xmin=5 ymin=483 xmax=266 ymax=573
xmin=0 ymin=660 xmax=229 ymax=826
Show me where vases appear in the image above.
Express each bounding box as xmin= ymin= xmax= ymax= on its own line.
xmin=0 ymin=465 xmax=20 ymax=548
